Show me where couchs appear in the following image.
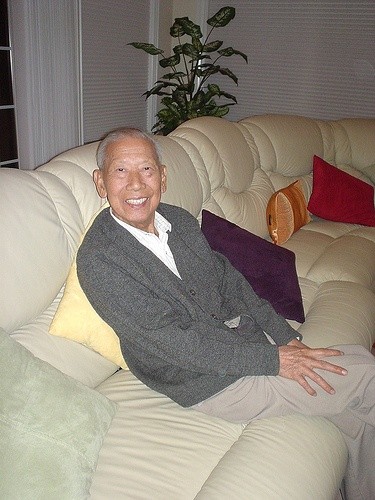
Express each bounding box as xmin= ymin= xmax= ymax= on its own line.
xmin=0 ymin=113 xmax=375 ymax=500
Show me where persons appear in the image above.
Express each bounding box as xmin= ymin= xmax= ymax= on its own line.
xmin=76 ymin=128 xmax=375 ymax=500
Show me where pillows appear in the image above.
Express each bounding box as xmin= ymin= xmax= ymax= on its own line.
xmin=306 ymin=154 xmax=375 ymax=227
xmin=0 ymin=328 xmax=117 ymax=500
xmin=201 ymin=208 xmax=305 ymax=323
xmin=265 ymin=179 xmax=314 ymax=246
xmin=47 ymin=200 xmax=130 ymax=371
